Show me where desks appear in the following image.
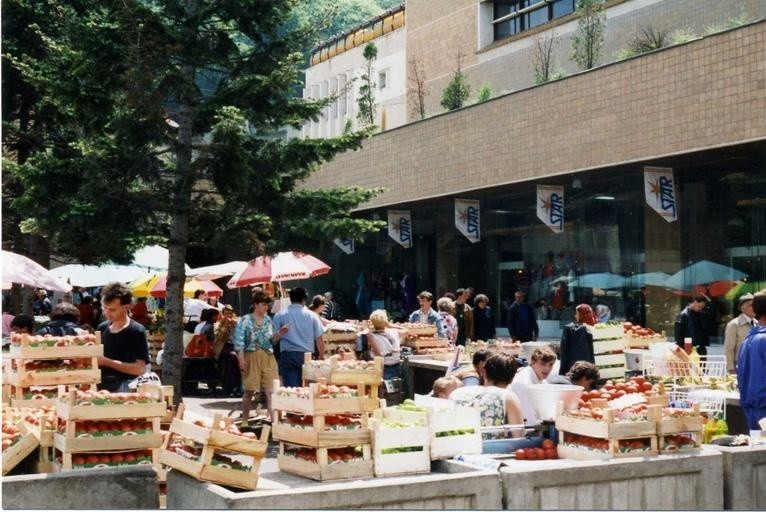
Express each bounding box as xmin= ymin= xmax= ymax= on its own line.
xmin=684 ymin=374 xmax=742 ymax=409
xmin=406 ymin=343 xmax=523 ymax=398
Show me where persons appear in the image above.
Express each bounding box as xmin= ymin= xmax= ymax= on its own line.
xmin=575 ymin=304 xmax=610 ymax=327
xmin=2 ymin=282 xmax=151 ymax=392
xmin=409 ymin=287 xmax=539 ymax=343
xmin=724 ymin=290 xmax=766 ymax=432
xmin=368 ymin=309 xmax=401 ymax=380
xmin=183 ymin=287 xmax=342 ymax=431
xmin=433 ymin=345 xmax=601 ymax=442
xmin=672 ymin=293 xmax=712 ymax=368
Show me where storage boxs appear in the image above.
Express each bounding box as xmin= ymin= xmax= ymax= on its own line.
xmin=272 ymin=378 xmax=375 ymax=480
xmin=302 ymin=350 xmax=383 ymax=406
xmin=3 ymin=408 xmax=40 ymax=476
xmin=8 ymin=329 xmax=103 ymax=407
xmin=557 ymin=400 xmax=657 ymax=460
xmin=622 ymin=320 xmax=666 ymax=380
xmin=393 ymin=321 xmax=448 ymax=355
xmin=368 ymin=410 xmax=430 ymax=475
xmin=381 ymin=396 xmax=481 ymax=458
xmin=321 ymin=321 xmax=362 ymax=360
xmin=163 ymin=401 xmax=271 ymax=491
xmin=55 ymin=387 xmax=172 ymax=484
xmin=658 ymin=396 xmax=701 ymax=454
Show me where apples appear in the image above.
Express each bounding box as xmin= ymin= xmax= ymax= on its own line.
xmin=0 ymin=329 xmax=255 ymax=494
xmin=592 ymin=320 xmax=661 ymax=370
xmin=279 ymin=322 xmax=474 ymax=463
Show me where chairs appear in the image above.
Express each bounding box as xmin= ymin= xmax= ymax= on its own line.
xmin=591 ymin=326 xmax=626 ymax=379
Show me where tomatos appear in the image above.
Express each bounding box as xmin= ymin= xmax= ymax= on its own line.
xmin=516 ymin=439 xmax=558 ymax=460
xmin=563 ymin=376 xmax=695 ymax=451
